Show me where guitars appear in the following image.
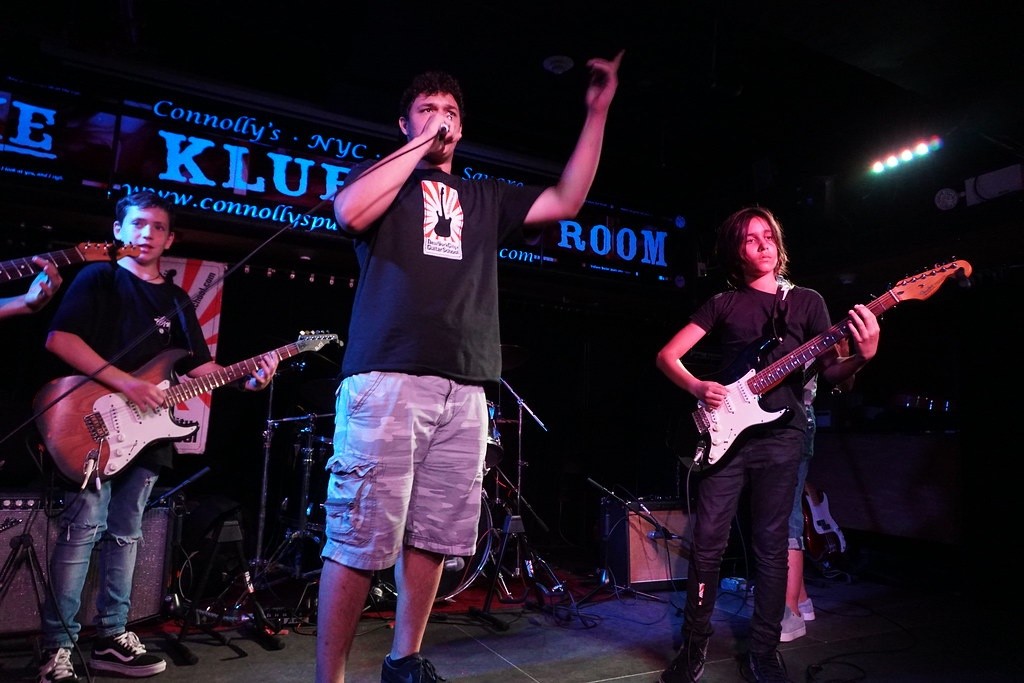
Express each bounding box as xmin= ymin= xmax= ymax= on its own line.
xmin=801 ymin=480 xmax=849 ymax=563
xmin=32 ymin=327 xmax=340 ymax=490
xmin=0 ymin=240 xmax=142 ymax=284
xmin=668 ymin=254 xmax=973 ymax=478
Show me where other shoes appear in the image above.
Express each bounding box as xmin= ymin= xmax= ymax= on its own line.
xmin=798 ymin=597 xmax=816 ymax=621
xmin=779 ymin=605 xmax=806 ymax=642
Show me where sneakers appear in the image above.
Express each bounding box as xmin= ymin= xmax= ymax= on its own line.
xmin=36 ymin=647 xmax=80 ymax=683
xmin=380 ymin=653 xmax=451 ymax=683
xmin=741 ymin=640 xmax=789 ymax=683
xmin=90 ymin=630 xmax=167 ymax=677
xmin=659 ymin=634 xmax=710 ymax=683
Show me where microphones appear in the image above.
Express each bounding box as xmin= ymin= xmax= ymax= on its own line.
xmin=438 ymin=123 xmax=449 ymax=139
xmin=647 ymin=530 xmax=681 ymax=540
xmin=443 ymin=556 xmax=464 ymax=571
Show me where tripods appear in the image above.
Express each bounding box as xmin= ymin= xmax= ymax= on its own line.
xmin=570 ymin=477 xmax=668 ymax=609
xmin=127 ymin=373 xmax=338 ymax=658
xmin=479 ymin=381 xmax=566 ymax=597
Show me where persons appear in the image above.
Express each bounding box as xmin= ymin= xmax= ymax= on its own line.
xmin=40 ymin=192 xmax=280 ymax=683
xmin=315 ymin=51 xmax=623 ymax=683
xmin=779 ymin=356 xmax=815 ymax=643
xmin=658 ymin=206 xmax=880 ymax=683
xmin=0 ymin=255 xmax=63 ymax=320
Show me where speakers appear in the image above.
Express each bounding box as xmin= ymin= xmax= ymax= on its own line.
xmin=611 ymin=499 xmax=695 ymax=587
xmin=0 ymin=493 xmax=174 ymax=643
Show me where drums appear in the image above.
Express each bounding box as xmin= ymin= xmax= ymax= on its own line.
xmin=485 ymin=399 xmax=506 ymax=469
xmin=272 ymin=432 xmax=334 ymax=533
xmin=375 ymin=492 xmax=495 ymax=601
